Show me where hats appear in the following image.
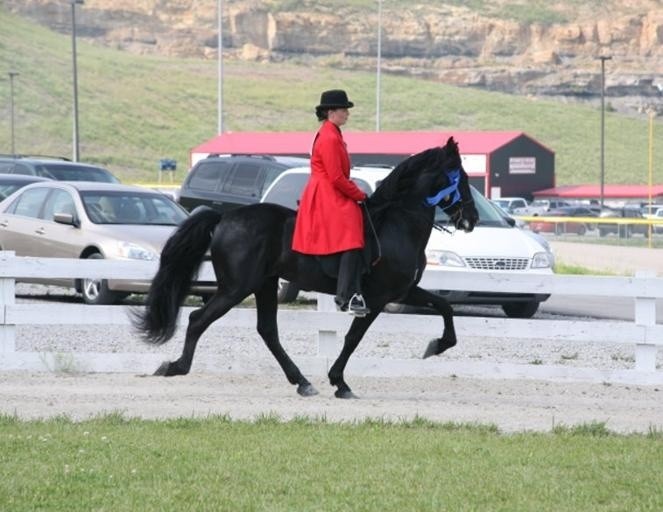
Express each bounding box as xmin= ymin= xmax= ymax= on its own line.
xmin=313 ymin=88 xmax=353 ymax=109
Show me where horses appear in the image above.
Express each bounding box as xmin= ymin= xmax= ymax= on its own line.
xmin=123 ymin=135 xmax=480 ymax=400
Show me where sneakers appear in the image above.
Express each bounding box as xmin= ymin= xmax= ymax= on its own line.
xmin=333 ymin=292 xmax=372 ymax=316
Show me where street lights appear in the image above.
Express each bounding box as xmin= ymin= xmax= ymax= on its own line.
xmin=7 ymin=70 xmax=20 ymax=154
xmin=592 ymin=54 xmax=615 ymax=205
xmin=71 ymin=1 xmax=91 ymax=162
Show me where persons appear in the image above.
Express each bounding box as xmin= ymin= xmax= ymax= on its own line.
xmin=290 ymin=90 xmax=369 ymax=316
